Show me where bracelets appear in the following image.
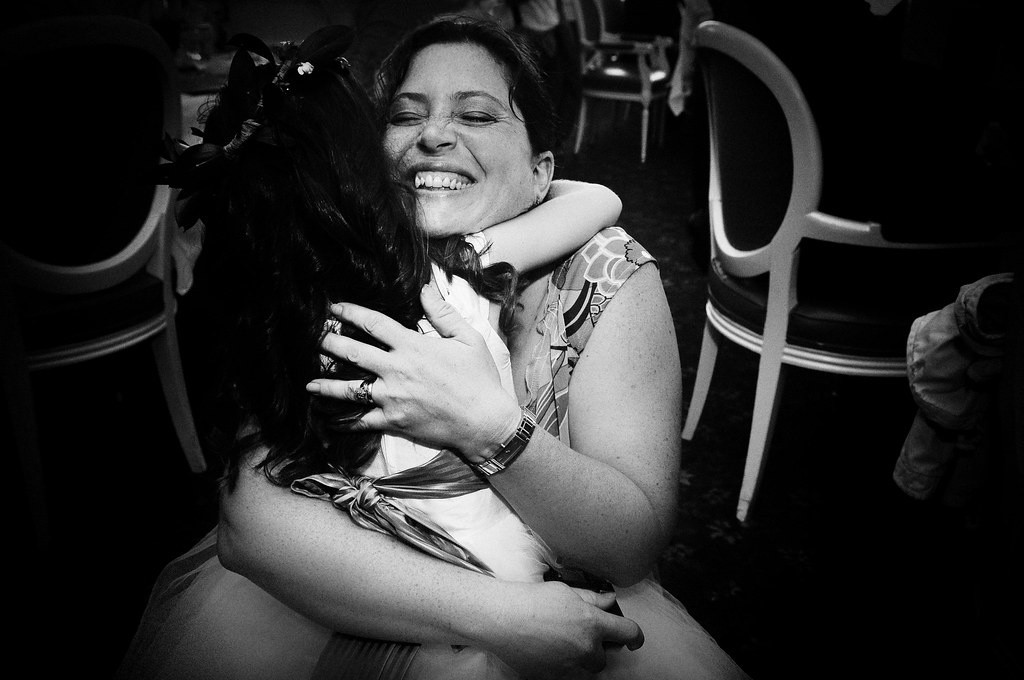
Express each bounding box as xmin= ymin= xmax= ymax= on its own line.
xmin=470 ymin=407 xmax=536 ymax=475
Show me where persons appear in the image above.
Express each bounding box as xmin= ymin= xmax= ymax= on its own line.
xmin=116 ymin=15 xmax=745 ymax=680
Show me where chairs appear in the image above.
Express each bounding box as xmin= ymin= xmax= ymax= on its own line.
xmin=570 ymin=0 xmax=674 ymax=163
xmin=0 ymin=15 xmax=208 ymax=476
xmin=679 ymin=20 xmax=964 ymax=523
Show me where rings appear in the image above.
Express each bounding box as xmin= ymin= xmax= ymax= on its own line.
xmin=356 ymin=381 xmax=375 ymax=406
